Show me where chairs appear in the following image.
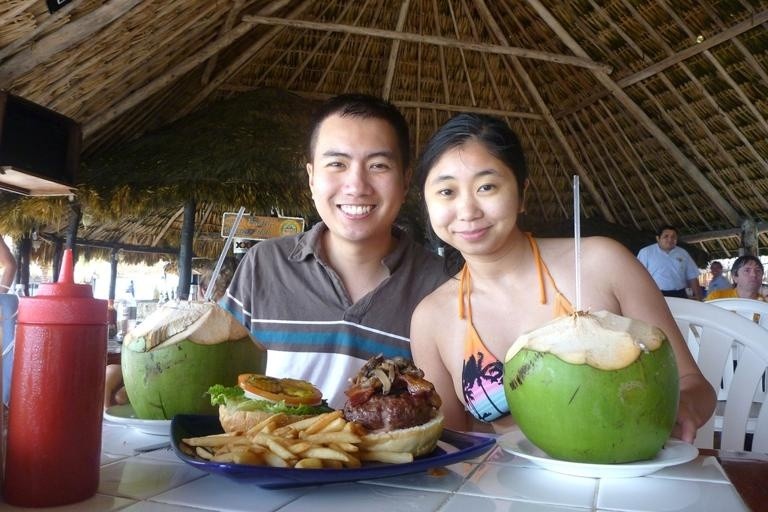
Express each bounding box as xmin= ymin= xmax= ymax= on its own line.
xmin=657 ymin=296 xmax=767 ymax=462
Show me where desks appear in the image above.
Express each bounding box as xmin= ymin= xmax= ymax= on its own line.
xmin=1 ymin=407 xmax=752 ymax=512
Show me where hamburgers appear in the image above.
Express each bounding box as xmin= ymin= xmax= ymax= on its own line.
xmin=204 ymin=375 xmax=337 ymax=433
xmin=344 ymin=354 xmax=445 ymax=457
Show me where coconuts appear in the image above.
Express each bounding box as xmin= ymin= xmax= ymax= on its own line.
xmin=121 ymin=299 xmax=267 ymax=418
xmin=503 ymin=309 xmax=680 ymax=463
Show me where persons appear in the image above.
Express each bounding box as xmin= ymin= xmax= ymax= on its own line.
xmin=408 ymin=112 xmax=718 ymax=445
xmin=706 ymin=261 xmax=734 ymax=293
xmin=197 ymin=256 xmax=239 ymax=303
xmin=635 ymin=225 xmax=703 ymax=303
xmin=0 ymin=235 xmax=18 ymax=294
xmin=102 ymin=92 xmax=459 ymax=414
xmin=703 ymin=254 xmax=767 ymax=392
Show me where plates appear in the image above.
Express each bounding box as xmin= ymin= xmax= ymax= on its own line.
xmin=104 ymin=404 xmax=172 ymax=435
xmin=169 ymin=411 xmax=499 ymax=492
xmin=494 ymin=431 xmax=698 ymax=477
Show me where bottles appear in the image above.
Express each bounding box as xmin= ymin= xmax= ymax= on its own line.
xmin=115 ymin=288 xmax=137 ymax=343
xmin=1 ymin=247 xmax=110 ymax=508
xmin=105 ymin=299 xmax=117 ymax=338
xmin=158 ymin=291 xmax=174 ymax=309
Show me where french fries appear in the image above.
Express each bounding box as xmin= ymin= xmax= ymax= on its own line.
xmin=180 ymin=410 xmax=362 ymax=470
